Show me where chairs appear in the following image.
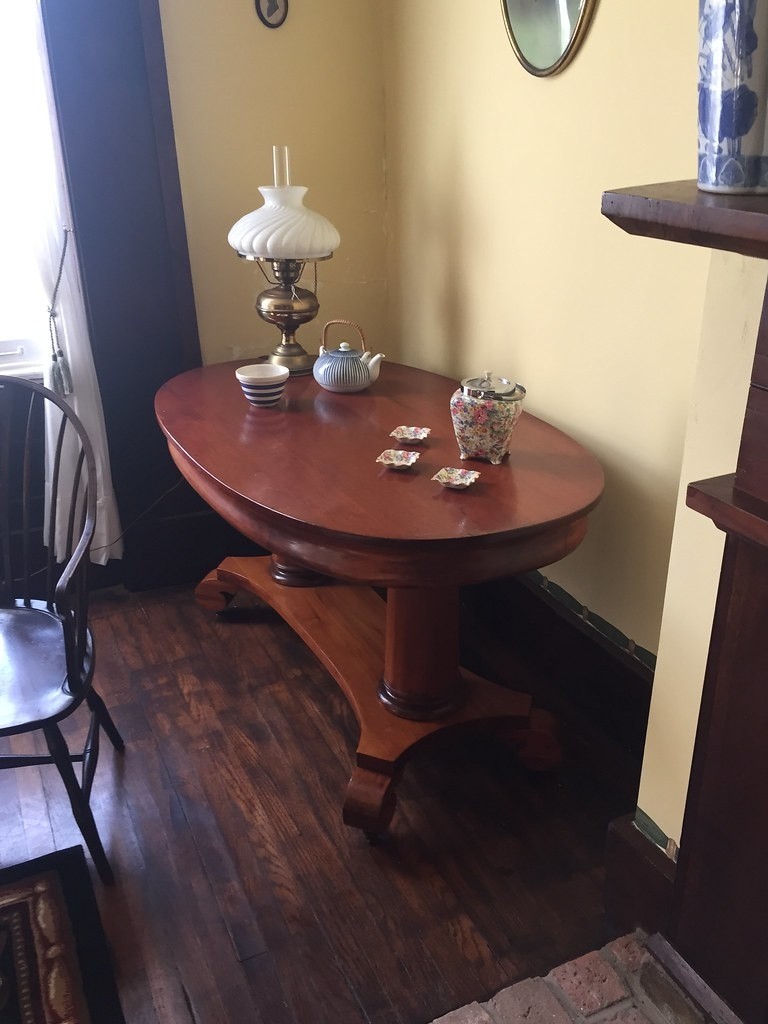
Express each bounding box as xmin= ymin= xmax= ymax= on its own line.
xmin=1 ymin=377 xmax=126 ymax=892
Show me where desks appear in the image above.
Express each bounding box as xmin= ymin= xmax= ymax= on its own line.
xmin=155 ymin=355 xmax=605 ymax=837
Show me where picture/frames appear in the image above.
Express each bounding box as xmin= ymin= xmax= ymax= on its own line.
xmin=254 ymin=0 xmax=289 ymax=28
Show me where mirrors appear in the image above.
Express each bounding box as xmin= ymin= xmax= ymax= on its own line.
xmin=499 ymin=0 xmax=598 ymax=78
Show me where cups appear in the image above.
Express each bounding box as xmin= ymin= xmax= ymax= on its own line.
xmin=235 ymin=364 xmax=290 ymax=407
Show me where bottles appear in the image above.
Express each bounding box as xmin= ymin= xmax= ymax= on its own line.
xmin=698 ymin=0 xmax=768 ymax=195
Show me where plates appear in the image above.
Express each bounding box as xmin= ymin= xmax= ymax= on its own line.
xmin=430 ymin=467 xmax=481 ymax=489
xmin=376 ymin=448 xmax=421 ymax=471
xmin=390 ymin=425 xmax=432 ymax=444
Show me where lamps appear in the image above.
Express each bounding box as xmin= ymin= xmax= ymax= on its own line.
xmin=226 ymin=144 xmax=342 ymax=377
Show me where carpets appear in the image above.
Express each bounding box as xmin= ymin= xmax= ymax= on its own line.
xmin=0 ymin=844 xmax=126 ymax=1024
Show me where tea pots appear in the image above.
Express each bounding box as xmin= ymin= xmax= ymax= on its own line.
xmin=313 ymin=320 xmax=386 ymax=393
xmin=450 ymin=370 xmax=527 ymax=465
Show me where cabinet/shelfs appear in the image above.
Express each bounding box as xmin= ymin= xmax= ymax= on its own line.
xmin=600 ymin=178 xmax=766 ymax=1024
